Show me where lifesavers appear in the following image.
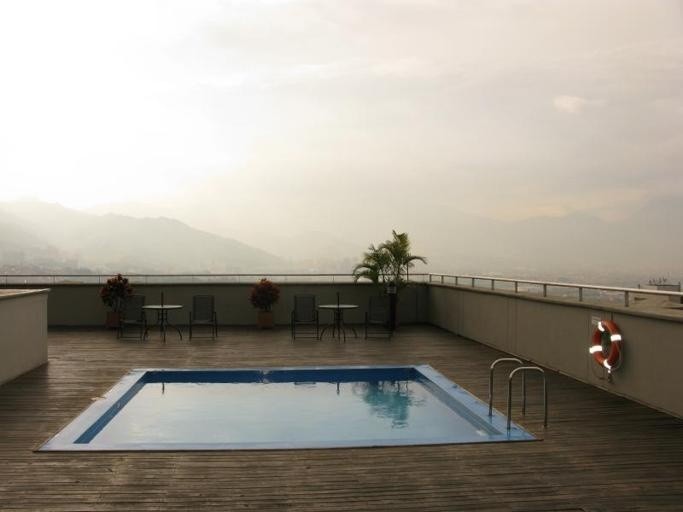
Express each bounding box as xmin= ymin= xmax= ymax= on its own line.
xmin=589 ymin=320 xmax=623 ymax=370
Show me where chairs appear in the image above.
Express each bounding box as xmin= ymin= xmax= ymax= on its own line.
xmin=114 ymin=293 xmax=148 ymax=341
xmin=287 ymin=293 xmax=320 ymax=341
xmin=188 ymin=294 xmax=219 ymax=340
xmin=364 ymin=294 xmax=398 ymax=341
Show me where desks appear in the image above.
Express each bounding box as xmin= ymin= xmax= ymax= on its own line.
xmin=141 ymin=303 xmax=184 ymax=345
xmin=318 ymin=302 xmax=360 ymax=341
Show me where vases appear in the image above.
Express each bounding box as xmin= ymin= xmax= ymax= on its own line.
xmin=256 ymin=310 xmax=274 ymax=329
xmin=104 ymin=311 xmax=120 ymax=329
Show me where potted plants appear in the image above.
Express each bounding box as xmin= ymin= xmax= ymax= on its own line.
xmin=351 ymin=229 xmax=427 ymax=331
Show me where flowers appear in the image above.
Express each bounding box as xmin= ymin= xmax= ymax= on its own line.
xmin=99 ymin=273 xmax=133 ymax=313
xmin=246 ymin=276 xmax=280 ymax=311
